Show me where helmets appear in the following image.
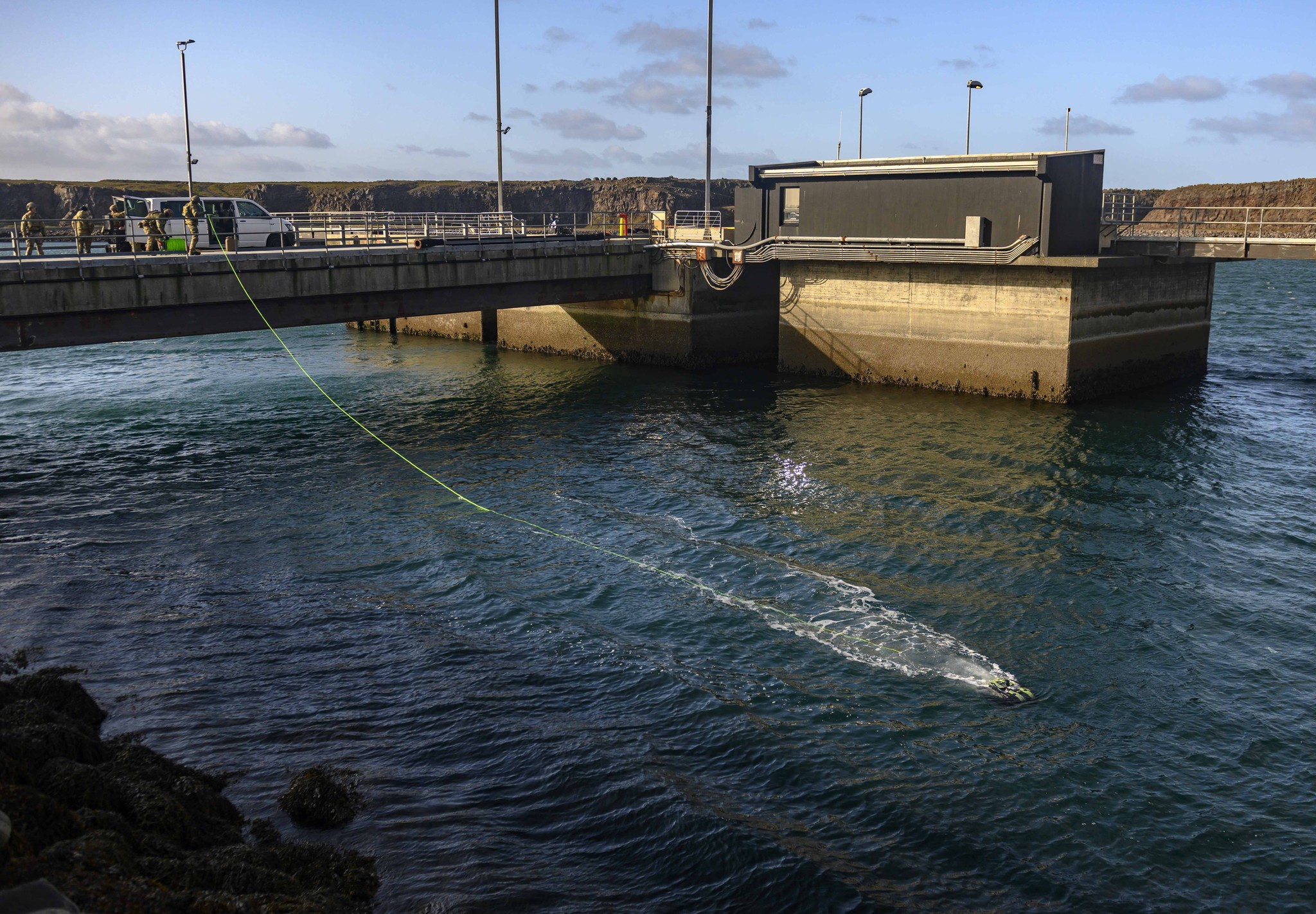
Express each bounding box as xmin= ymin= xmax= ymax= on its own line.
xmin=110 ymin=205 xmax=118 ymax=210
xmin=26 ymin=202 xmax=35 ymax=211
xmin=164 ymin=209 xmax=173 ymax=217
xmin=191 ymin=195 xmax=200 ymax=204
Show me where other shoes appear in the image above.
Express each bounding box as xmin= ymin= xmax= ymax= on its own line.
xmin=188 ymin=251 xmax=201 ymax=255
xmin=146 ymin=250 xmax=156 ymax=256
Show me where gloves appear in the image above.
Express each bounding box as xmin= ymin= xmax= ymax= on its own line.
xmin=163 ymin=236 xmax=169 ymax=240
xmin=23 ymin=235 xmax=27 ymax=241
xmin=205 ymin=211 xmax=208 ymax=214
xmin=42 ymin=235 xmax=46 ymax=240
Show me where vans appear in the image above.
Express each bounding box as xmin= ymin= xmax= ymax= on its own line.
xmin=110 ymin=195 xmax=295 ymax=249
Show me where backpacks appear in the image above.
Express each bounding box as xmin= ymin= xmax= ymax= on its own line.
xmin=182 ymin=203 xmax=195 ymax=217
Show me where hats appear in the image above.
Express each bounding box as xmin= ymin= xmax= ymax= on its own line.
xmin=79 ymin=206 xmax=87 ymax=211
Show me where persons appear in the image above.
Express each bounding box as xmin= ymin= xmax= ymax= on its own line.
xmin=142 ymin=208 xmax=174 ymax=256
xmin=107 ymin=204 xmax=135 ymax=243
xmin=184 ymin=195 xmax=210 ymax=255
xmin=72 ymin=206 xmax=94 ymax=254
xmin=21 ymin=203 xmax=47 ymax=256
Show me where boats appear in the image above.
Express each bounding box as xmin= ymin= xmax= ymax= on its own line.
xmin=985 ymin=676 xmax=1036 ymax=702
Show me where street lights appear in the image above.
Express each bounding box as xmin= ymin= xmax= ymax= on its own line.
xmin=176 ymin=39 xmax=196 ymax=198
xmin=858 ymin=87 xmax=872 ymax=158
xmin=965 ymin=80 xmax=983 ymax=155
